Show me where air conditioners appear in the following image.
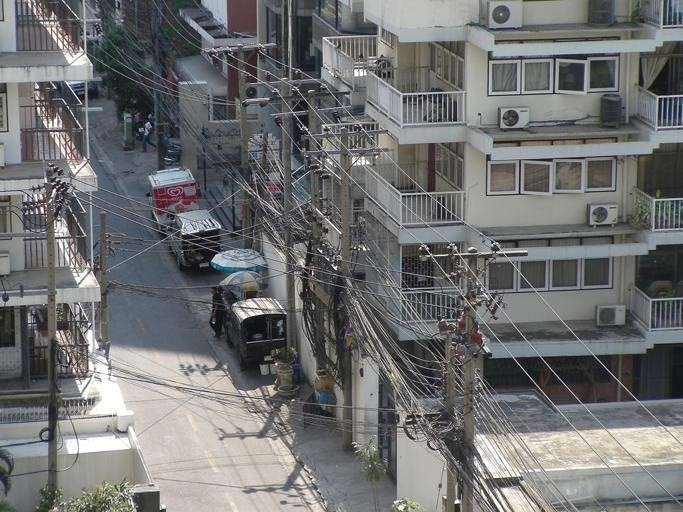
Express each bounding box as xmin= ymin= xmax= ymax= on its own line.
xmin=1 ymin=144 xmax=4 ymax=166
xmin=0 ymin=252 xmax=10 ymax=277
xmin=486 ymin=0 xmax=524 ymax=29
xmin=597 ymin=305 xmax=627 ymax=327
xmin=589 ymin=204 xmax=619 ymax=226
xmin=243 ymin=83 xmax=258 ymax=98
xmin=499 ymin=107 xmax=530 ymax=130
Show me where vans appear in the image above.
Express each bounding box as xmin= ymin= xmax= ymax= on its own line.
xmin=163 ymin=210 xmax=224 ymax=270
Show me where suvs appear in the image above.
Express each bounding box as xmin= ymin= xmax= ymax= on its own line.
xmin=65 ymin=79 xmax=100 ymax=101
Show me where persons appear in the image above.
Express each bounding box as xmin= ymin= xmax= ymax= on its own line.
xmin=209 ymin=287 xmax=225 ymax=338
xmin=133 ymin=111 xmax=156 ymax=152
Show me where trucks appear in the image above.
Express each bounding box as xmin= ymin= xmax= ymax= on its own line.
xmin=143 ymin=166 xmax=202 ymax=231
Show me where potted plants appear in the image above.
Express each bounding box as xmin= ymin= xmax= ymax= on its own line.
xmin=271 ymin=346 xmax=297 ymax=385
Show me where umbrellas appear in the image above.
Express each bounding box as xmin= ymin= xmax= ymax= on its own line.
xmin=211 ymin=249 xmax=268 ymax=298
